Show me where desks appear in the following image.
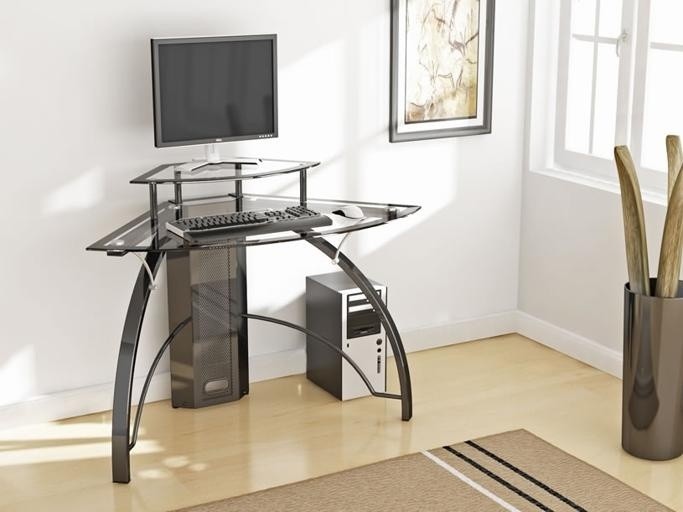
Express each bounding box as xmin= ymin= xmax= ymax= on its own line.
xmin=86 ymin=158 xmax=421 ymax=484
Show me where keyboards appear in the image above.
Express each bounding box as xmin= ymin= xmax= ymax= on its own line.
xmin=166 ymin=205 xmax=332 ymax=243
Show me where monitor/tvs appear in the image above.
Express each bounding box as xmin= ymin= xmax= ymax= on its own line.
xmin=151 ymin=34 xmax=278 ymax=172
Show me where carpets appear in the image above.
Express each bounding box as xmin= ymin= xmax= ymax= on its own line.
xmin=163 ymin=428 xmax=677 ymax=512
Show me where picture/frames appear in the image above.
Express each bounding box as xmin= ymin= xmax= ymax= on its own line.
xmin=390 ymin=0 xmax=495 ymax=143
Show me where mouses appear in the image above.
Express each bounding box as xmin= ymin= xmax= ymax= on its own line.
xmin=332 ymin=205 xmax=364 ymax=219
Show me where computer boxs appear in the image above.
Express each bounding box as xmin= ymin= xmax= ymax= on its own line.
xmin=306 ymin=271 xmax=388 ymax=402
